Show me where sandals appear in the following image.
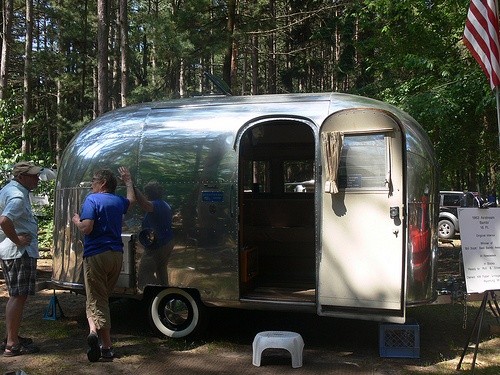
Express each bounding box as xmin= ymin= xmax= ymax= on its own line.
xmin=0 ymin=336 xmax=33 ymax=350
xmin=3 ymin=343 xmax=39 ymax=357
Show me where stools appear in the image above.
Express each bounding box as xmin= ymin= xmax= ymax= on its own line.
xmin=252 ymin=331 xmax=304 ymax=368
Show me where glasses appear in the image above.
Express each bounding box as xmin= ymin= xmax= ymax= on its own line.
xmin=24 ymin=173 xmax=40 ymax=179
xmin=91 ymin=177 xmax=102 ymax=182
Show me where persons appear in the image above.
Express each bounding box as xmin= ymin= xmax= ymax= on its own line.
xmin=72 ymin=166 xmax=135 ymax=360
xmin=458 ymin=186 xmax=495 ymax=209
xmin=0 ymin=162 xmax=44 ymax=357
xmin=135 ymin=181 xmax=176 ymax=286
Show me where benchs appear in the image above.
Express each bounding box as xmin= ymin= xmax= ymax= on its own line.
xmin=244 ymin=198 xmax=314 ymax=272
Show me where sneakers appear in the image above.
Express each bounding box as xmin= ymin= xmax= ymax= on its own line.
xmin=87 ymin=331 xmax=101 ymax=362
xmin=99 ymin=344 xmax=113 ymax=361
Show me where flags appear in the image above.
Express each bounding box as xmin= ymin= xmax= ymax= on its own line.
xmin=463 ymin=0 xmax=500 ymax=90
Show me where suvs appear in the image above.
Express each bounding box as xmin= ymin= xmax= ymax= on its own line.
xmin=439 ymin=188 xmax=482 ymax=241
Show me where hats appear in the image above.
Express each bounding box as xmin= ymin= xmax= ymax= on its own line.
xmin=13 ymin=162 xmax=42 ymax=176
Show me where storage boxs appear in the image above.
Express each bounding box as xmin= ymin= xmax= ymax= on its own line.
xmin=378 ymin=317 xmax=421 ymax=358
